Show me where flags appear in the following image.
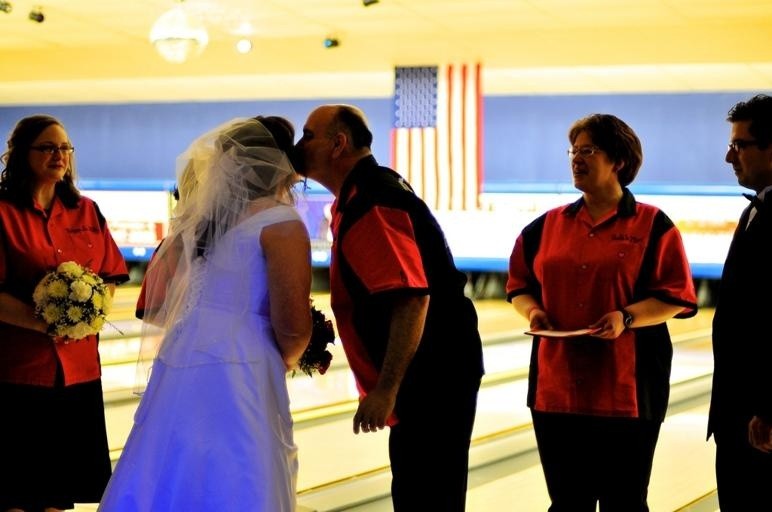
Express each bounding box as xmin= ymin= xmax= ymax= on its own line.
xmin=390 ymin=63 xmax=482 ymax=211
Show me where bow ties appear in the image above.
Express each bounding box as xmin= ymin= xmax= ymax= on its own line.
xmin=742 ymin=192 xmax=764 ymax=211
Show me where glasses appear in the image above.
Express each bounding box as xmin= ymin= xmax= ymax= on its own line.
xmin=728 ymin=141 xmax=754 ymax=152
xmin=29 ymin=144 xmax=75 ymax=155
xmin=567 ymin=146 xmax=600 ymax=157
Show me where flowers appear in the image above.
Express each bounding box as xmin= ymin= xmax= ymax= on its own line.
xmin=288 ymin=301 xmax=332 ymax=378
xmin=33 ymin=261 xmax=111 ymax=343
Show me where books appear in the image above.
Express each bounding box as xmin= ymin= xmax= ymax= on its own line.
xmin=524 ymin=325 xmax=603 ymax=338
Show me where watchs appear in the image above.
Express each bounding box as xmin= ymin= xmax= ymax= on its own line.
xmin=619 ymin=306 xmax=633 ymax=332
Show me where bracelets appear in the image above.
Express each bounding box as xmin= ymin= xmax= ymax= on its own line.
xmin=526 ymin=304 xmax=541 ymax=313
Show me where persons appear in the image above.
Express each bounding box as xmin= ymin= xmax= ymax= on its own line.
xmin=135 ymin=189 xmax=186 ymax=336
xmin=705 ymin=94 xmax=771 ymax=512
xmin=1 ymin=112 xmax=130 ymax=511
xmin=292 ymin=104 xmax=485 ymax=512
xmin=95 ymin=115 xmax=312 ymax=512
xmin=504 ymin=114 xmax=699 ymax=512
